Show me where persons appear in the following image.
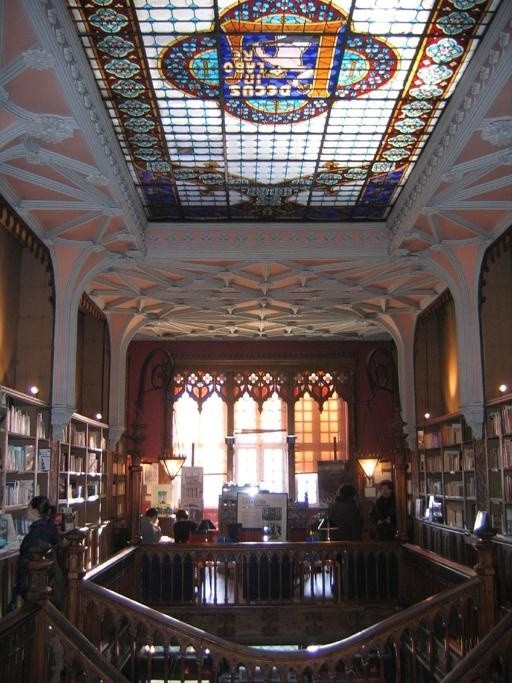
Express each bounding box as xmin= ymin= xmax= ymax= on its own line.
xmin=328 ymin=481 xmax=364 ymax=593
xmin=172 ymin=509 xmax=198 ymax=542
xmin=8 ymin=495 xmax=65 ymax=609
xmin=140 ymin=508 xmax=163 ymax=544
xmin=369 ymin=479 xmax=399 ymax=598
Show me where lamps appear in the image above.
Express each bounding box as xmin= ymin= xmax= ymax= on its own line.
xmin=354 ymin=343 xmax=407 ymax=543
xmin=128 ymin=344 xmax=191 ymax=542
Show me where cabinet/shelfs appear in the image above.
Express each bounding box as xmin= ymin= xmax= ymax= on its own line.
xmin=476 ymin=389 xmax=512 ymax=613
xmin=407 ymin=409 xmax=477 ymax=568
xmin=0 ymin=382 xmax=55 ymax=572
xmin=56 ymin=410 xmax=105 ymax=578
xmin=106 ymin=447 xmax=129 ymax=556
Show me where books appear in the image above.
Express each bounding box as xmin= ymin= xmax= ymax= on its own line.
xmin=0 ymin=405 xmax=127 ymax=549
xmin=405 ymin=405 xmax=511 ymax=535
